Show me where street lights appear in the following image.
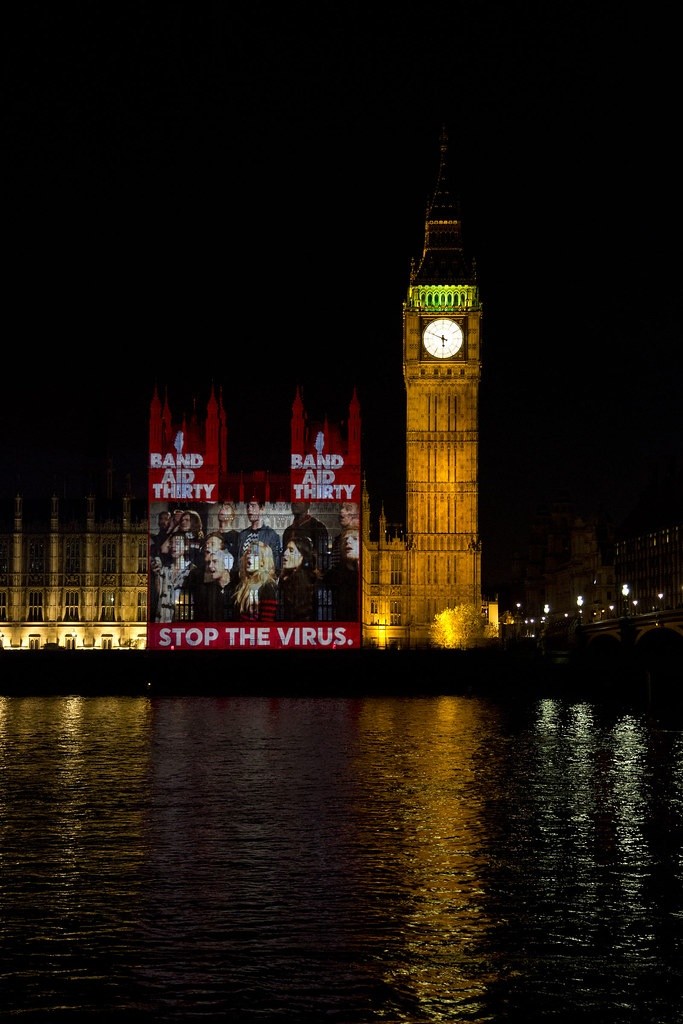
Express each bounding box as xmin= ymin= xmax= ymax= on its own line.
xmin=576 ymin=595 xmax=585 ymax=624
xmin=658 ymin=592 xmax=664 ymax=610
xmin=544 ymin=603 xmax=550 ymax=629
xmin=524 ymin=619 xmax=535 ymax=638
xmin=622 ymin=582 xmax=631 ymax=615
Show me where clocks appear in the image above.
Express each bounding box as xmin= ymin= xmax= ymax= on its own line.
xmin=421 ymin=317 xmax=465 ymax=359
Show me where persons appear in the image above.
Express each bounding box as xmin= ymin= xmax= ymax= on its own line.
xmin=147 ymin=500 xmax=358 ymax=623
